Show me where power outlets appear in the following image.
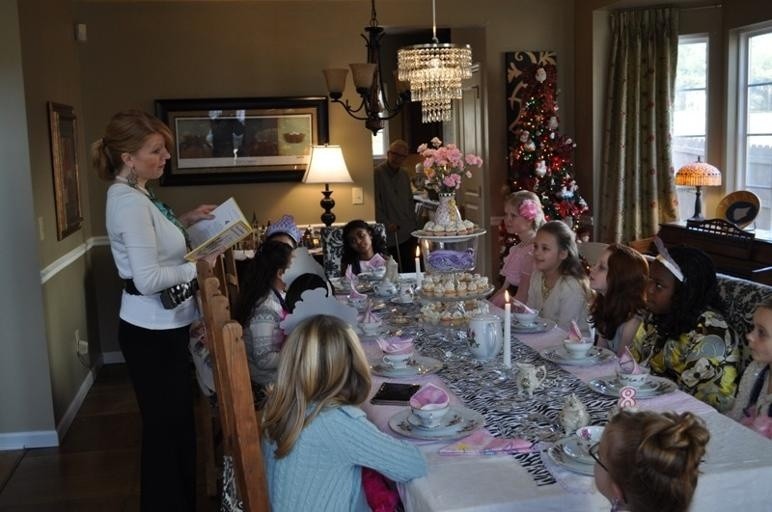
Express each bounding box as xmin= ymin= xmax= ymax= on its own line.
xmin=74 ymin=331 xmax=87 ymax=356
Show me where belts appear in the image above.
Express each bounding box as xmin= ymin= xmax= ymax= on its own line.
xmin=122 ymin=278 xmax=199 ymax=308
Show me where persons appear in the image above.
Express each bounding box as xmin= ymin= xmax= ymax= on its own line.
xmin=489 ymin=190 xmax=772 ymax=440
xmin=587 ymin=411 xmax=713 ymax=512
xmin=86 ymin=107 xmax=230 ymax=511
xmin=228 ymin=216 xmax=423 ymax=511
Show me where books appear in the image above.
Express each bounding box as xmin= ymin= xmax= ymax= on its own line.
xmin=183 ymin=197 xmax=252 ymax=263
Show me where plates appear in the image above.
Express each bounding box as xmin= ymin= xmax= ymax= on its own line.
xmin=330 ymin=271 xmax=444 ymax=378
xmin=409 ymin=228 xmax=488 ymax=246
xmin=585 ymin=375 xmax=681 ymax=397
xmin=413 ymin=282 xmax=495 ymax=302
xmin=547 ymin=435 xmax=600 ymax=477
xmin=716 ymin=190 xmax=761 ymax=233
xmin=386 ymin=403 xmax=487 ymax=441
xmin=501 ymin=314 xmax=558 ymax=334
xmin=540 ymin=343 xmax=614 ymax=365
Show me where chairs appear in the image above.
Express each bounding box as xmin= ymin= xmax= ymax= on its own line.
xmin=196 ymin=246 xmax=274 ymax=512
xmin=572 ymin=241 xmax=772 ymax=384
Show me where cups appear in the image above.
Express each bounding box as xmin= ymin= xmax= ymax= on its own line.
xmin=575 ymin=425 xmax=607 ymax=452
xmin=516 ymin=361 xmax=548 ymax=397
xmin=406 ymin=404 xmax=452 ymax=428
xmin=614 ymin=366 xmax=651 ymax=390
xmin=342 ymin=265 xmax=415 ymax=368
xmin=512 ymin=311 xmax=539 ymax=325
xmin=560 ymin=338 xmax=594 ymax=360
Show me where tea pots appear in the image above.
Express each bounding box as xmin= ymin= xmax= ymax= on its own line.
xmin=466 ymin=302 xmax=506 ymax=362
xmin=371 ymin=276 xmax=398 ymax=297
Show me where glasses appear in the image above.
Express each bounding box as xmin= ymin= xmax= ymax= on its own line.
xmin=590 ymin=443 xmax=627 ymax=507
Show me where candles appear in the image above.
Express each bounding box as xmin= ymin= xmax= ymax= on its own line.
xmin=414 ymin=244 xmax=423 ymax=289
xmin=503 ymin=289 xmax=512 ymax=370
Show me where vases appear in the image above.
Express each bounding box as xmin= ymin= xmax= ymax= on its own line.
xmin=431 ymin=192 xmax=463 ymax=229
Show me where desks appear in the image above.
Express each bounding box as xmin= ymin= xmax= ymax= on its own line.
xmin=658 ymin=220 xmax=772 ymax=286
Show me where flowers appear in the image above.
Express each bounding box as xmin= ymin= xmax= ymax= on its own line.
xmin=414 ymin=137 xmax=483 ymax=196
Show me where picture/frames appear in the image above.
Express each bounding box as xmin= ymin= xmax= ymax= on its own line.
xmin=153 ymin=95 xmax=329 ymax=188
xmin=46 ymin=100 xmax=84 ymax=241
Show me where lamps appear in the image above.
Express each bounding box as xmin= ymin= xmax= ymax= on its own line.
xmin=675 ymin=154 xmax=723 ymax=230
xmin=322 ymin=1 xmax=411 ymax=136
xmin=301 ymin=144 xmax=355 ymax=228
xmin=395 ymin=0 xmax=472 ymax=125
